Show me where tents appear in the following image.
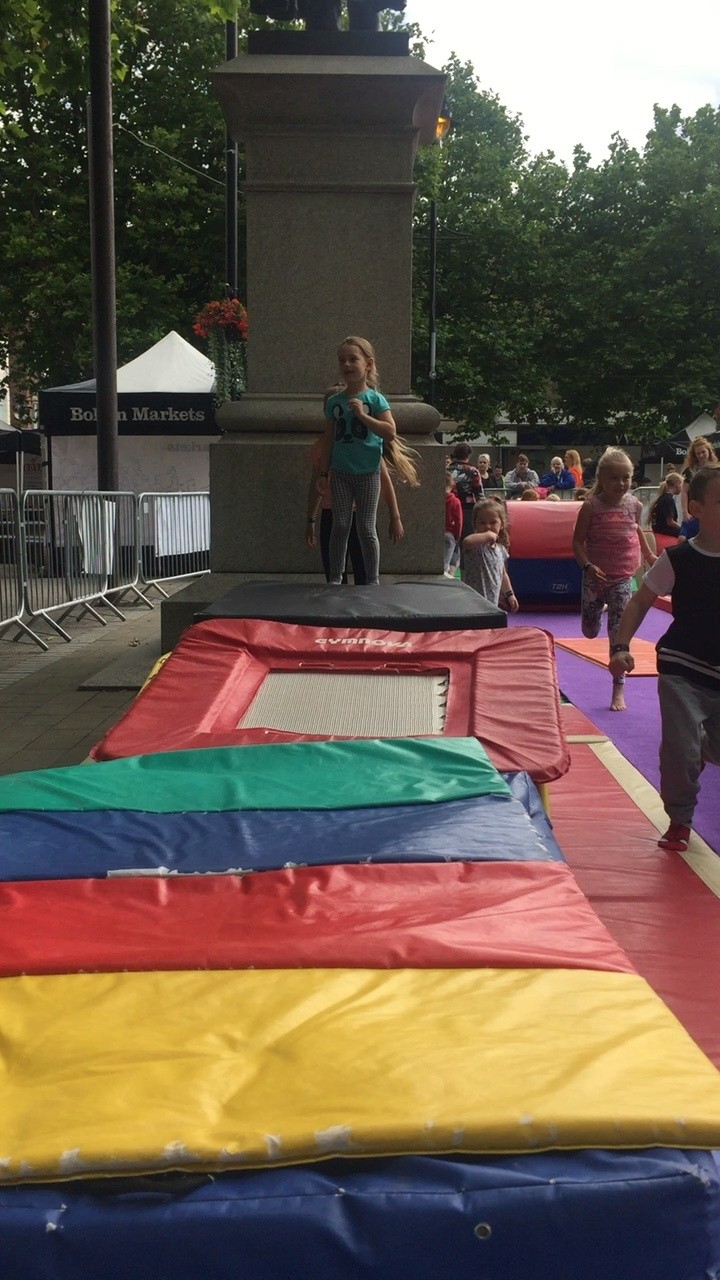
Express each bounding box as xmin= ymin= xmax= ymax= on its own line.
xmin=639 ymin=412 xmax=719 ymax=481
xmin=39 ymin=331 xmax=217 ymax=577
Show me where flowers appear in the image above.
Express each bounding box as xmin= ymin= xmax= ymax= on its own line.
xmin=192 ymin=296 xmax=249 ymax=408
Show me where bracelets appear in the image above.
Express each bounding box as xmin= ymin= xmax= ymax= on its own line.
xmin=320 ymin=471 xmax=329 ymax=478
xmin=503 ymin=590 xmax=514 ymax=599
xmin=643 ymin=551 xmax=653 ymax=558
xmin=582 ymin=562 xmax=592 ymax=573
xmin=611 ymin=643 xmax=629 ymax=654
xmin=309 ymin=518 xmax=316 ymax=523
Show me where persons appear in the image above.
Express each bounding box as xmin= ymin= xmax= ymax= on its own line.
xmin=573 ymin=449 xmax=659 ymax=711
xmin=443 ymin=437 xmax=720 ymax=613
xmin=609 ymin=465 xmax=720 ymax=852
xmin=306 ymin=337 xmax=420 ymax=585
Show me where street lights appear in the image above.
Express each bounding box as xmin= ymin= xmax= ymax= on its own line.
xmin=424 ymin=111 xmax=454 ymax=404
